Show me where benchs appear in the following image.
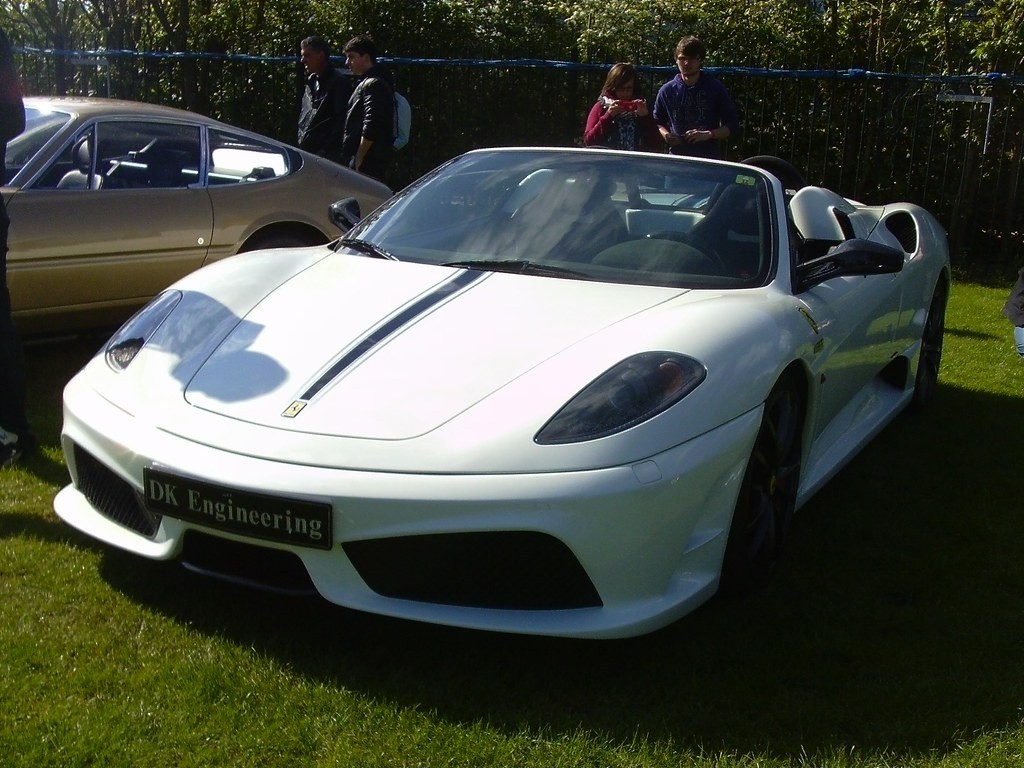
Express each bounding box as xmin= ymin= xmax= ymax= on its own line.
xmin=625 ymin=205 xmax=707 ymax=234
xmin=110 ymin=159 xmax=256 ymax=184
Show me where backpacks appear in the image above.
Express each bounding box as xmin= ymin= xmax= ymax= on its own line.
xmin=392 ymin=92 xmax=411 ymax=151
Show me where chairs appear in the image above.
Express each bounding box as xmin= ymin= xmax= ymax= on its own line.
xmin=54 ymin=136 xmax=106 ymax=190
xmin=681 ymin=184 xmax=768 ymax=274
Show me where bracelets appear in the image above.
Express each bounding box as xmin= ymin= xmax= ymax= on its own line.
xmin=711 ymin=129 xmax=715 ymax=138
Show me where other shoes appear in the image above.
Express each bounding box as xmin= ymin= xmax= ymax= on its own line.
xmin=0 ymin=422 xmax=29 ymax=468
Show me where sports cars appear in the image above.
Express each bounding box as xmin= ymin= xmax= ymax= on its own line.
xmin=53 ymin=145 xmax=953 ymax=640
xmin=1 ymin=96 xmax=397 ymax=337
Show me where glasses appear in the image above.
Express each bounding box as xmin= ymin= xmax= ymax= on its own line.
xmin=676 ymin=56 xmax=701 ymax=65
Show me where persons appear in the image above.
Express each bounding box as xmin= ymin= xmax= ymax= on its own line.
xmin=584 ymin=62 xmax=663 ymax=153
xmin=298 ymin=36 xmax=395 ymax=189
xmin=0 ymin=25 xmax=38 ymax=470
xmin=652 ymin=35 xmax=740 ymax=159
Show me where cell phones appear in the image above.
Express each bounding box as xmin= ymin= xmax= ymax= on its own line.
xmin=618 ymin=101 xmax=635 ymax=112
xmin=678 ymin=134 xmax=687 ymax=145
